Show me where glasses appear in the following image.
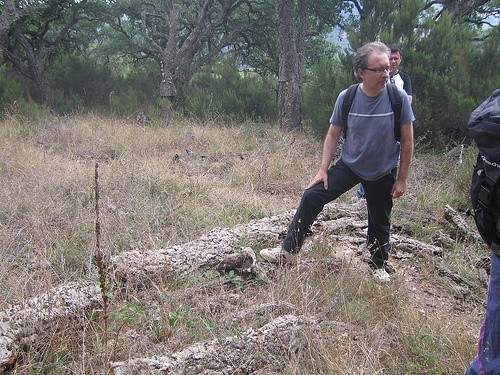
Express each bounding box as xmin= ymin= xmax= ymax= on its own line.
xmin=365 ymin=67 xmax=393 ymax=73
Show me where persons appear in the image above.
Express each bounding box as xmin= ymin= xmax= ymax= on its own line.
xmin=463 ymin=87 xmax=500 ymax=374
xmin=356 ymin=43 xmax=413 ymax=202
xmin=258 ymin=42 xmax=414 ymax=281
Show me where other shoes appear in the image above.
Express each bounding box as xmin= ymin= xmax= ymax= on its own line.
xmin=261 ymin=244 xmax=298 ymax=267
xmin=371 ymin=268 xmax=389 ymax=284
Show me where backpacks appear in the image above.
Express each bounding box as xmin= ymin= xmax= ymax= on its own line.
xmin=468 ymin=88 xmax=500 ymax=257
